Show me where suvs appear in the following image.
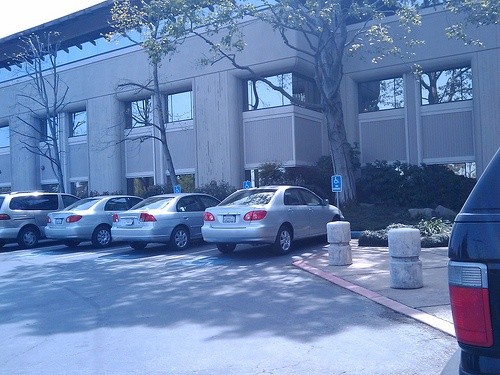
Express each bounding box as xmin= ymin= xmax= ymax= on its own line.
xmin=0 ymin=191 xmax=83 ymax=248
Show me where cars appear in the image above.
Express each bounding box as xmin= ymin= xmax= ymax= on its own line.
xmin=448 ymin=145 xmax=500 ymax=375
xmin=111 ymin=192 xmax=222 ymax=250
xmin=45 ymin=195 xmax=146 ymax=249
xmin=202 ymin=184 xmax=345 ymax=254
